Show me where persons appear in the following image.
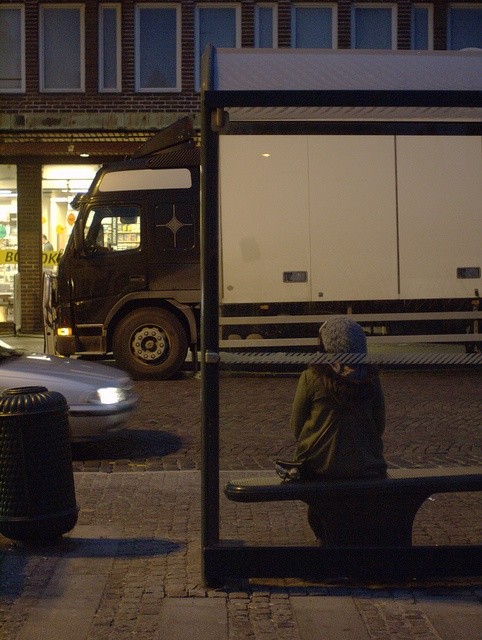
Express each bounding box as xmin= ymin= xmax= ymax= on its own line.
xmin=290 ymin=316 xmax=388 ymax=548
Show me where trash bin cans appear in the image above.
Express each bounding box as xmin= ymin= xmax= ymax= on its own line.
xmin=0 ymin=386 xmax=78 ymax=540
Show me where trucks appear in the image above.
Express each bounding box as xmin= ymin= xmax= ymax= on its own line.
xmin=46 ymin=39 xmax=481 ymax=381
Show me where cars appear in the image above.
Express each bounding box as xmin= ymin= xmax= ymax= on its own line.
xmin=1 ymin=339 xmax=141 ymax=439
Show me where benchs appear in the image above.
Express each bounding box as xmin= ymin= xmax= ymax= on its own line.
xmin=222 ymin=467 xmax=482 ymax=583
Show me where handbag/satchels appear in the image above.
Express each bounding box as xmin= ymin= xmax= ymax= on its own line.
xmin=276 ymin=458 xmax=310 ymax=485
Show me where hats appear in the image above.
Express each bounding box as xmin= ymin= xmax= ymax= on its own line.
xmin=319 ymin=317 xmax=367 ymax=356
